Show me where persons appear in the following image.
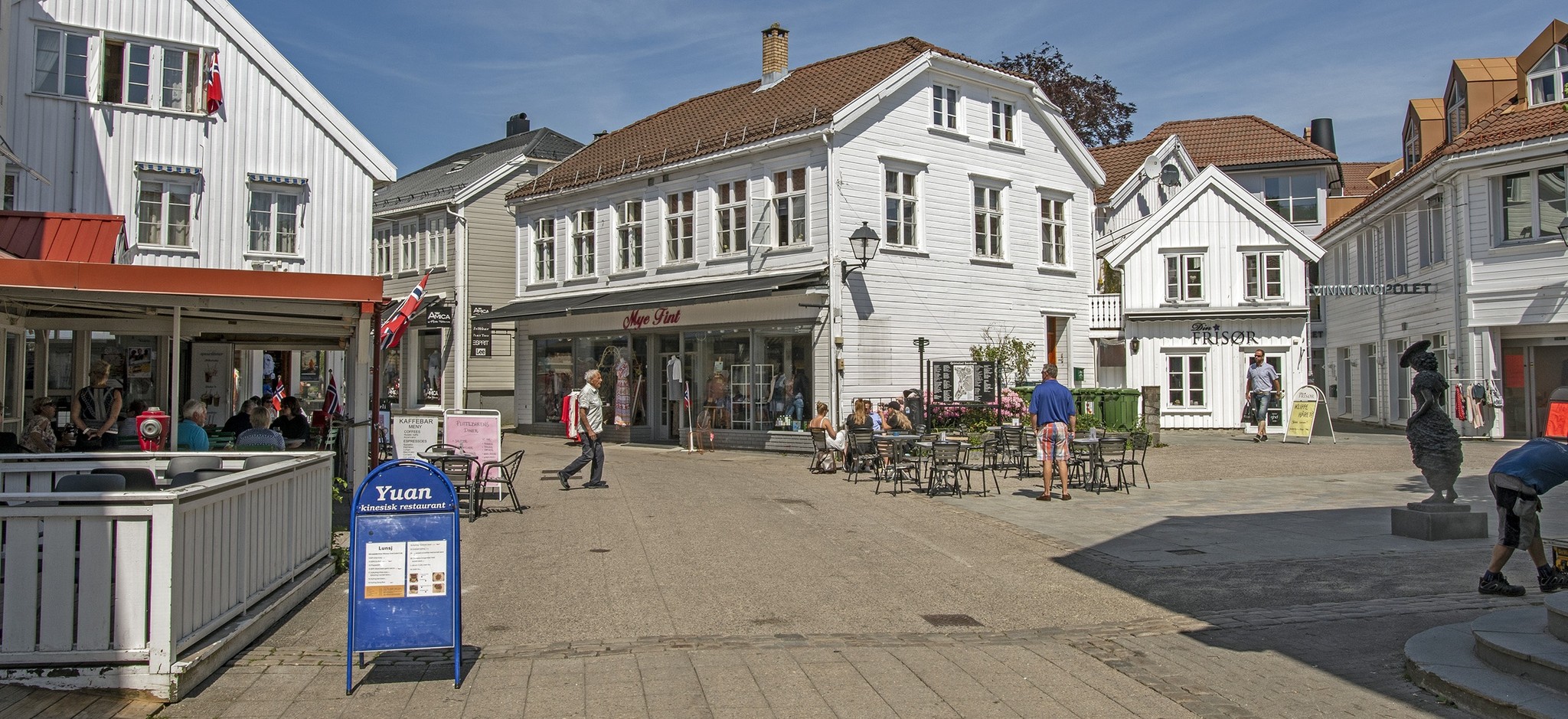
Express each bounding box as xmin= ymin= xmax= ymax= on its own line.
xmin=1478 ymin=438 xmax=1568 ymax=597
xmin=1245 ymin=349 xmax=1282 ymax=443
xmin=70 ymin=360 xmax=122 ymax=451
xmin=1400 ymin=340 xmax=1463 ymax=502
xmin=19 ymin=397 xmax=69 ymax=463
xmin=556 ymin=369 xmax=610 ymax=489
xmin=846 ymin=397 xmax=873 ymax=472
xmin=176 ymin=399 xmax=234 ymax=452
xmin=1029 ymin=364 xmax=1077 ymax=499
xmin=877 ymin=401 xmax=913 ymax=467
xmin=119 ymin=399 xmax=148 ymax=439
xmin=809 ymin=401 xmax=848 ymax=460
xmin=862 ymin=400 xmax=884 ymax=436
xmin=215 ymin=395 xmax=310 ymax=453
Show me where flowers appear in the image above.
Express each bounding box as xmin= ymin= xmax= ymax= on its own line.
xmin=798 ymin=234 xmax=804 ymax=239
xmin=724 ymin=245 xmax=729 ymax=249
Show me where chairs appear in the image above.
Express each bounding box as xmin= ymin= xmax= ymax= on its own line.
xmin=309 ymin=426 xmax=339 ymax=451
xmin=0 ymin=456 xmax=297 ymax=594
xmin=421 ymin=443 xmax=465 ymax=498
xmin=809 ymin=424 xmax=1150 ymax=498
xmin=478 ymin=449 xmax=525 ymax=515
xmin=430 ymin=455 xmax=481 ymax=522
xmin=116 ymin=431 xmax=277 ymax=451
xmin=0 ymin=432 xmax=44 ymax=462
xmin=687 ymin=410 xmax=714 ymax=455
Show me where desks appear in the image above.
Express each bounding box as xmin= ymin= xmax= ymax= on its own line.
xmin=417 ymin=451 xmax=479 ymax=480
xmin=1073 ymin=439 xmax=1111 ymax=489
xmin=873 ymin=434 xmax=920 ymax=494
xmin=913 ymin=441 xmax=972 ymax=492
xmin=285 ymin=438 xmax=305 ymax=449
xmin=987 ymin=426 xmax=1026 ymax=469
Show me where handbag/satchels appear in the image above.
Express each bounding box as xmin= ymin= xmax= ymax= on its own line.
xmin=1241 ymin=401 xmax=1257 ymax=423
xmin=816 ymin=451 xmax=836 ymax=473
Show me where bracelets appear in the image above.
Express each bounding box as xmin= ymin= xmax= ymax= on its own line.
xmin=1276 ymin=393 xmax=1280 ymax=396
xmin=1032 ymin=427 xmax=1036 ymax=431
xmin=82 ymin=427 xmax=90 ymax=434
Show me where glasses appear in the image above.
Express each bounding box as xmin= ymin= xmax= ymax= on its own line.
xmin=823 ymin=409 xmax=829 ymax=412
xmin=1255 ymin=355 xmax=1263 ymax=357
xmin=43 ymin=403 xmax=55 ymax=407
xmin=265 ymin=400 xmax=273 ymax=404
xmin=866 ymin=408 xmax=871 ymax=410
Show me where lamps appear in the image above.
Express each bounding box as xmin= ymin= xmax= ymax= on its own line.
xmin=1130 ymin=337 xmax=1140 ymax=353
xmin=841 ymin=221 xmax=881 ymax=284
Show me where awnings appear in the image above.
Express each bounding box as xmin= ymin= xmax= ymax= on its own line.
xmin=471 ymin=267 xmax=831 ymax=326
xmin=0 ymin=144 xmax=51 ymax=186
xmin=1123 ymin=305 xmax=1308 ymax=322
xmin=134 ymin=161 xmax=202 ymax=218
xmin=375 ymin=293 xmax=442 ymax=331
xmin=247 ymin=172 xmax=308 ymax=226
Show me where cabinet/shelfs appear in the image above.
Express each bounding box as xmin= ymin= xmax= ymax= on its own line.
xmin=731 ymin=365 xmax=774 ymax=431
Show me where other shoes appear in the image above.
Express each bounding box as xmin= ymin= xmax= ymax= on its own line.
xmin=1063 ymin=493 xmax=1071 ymax=501
xmin=879 ymin=467 xmax=893 ymax=472
xmin=864 ymin=467 xmax=870 ymax=472
xmin=842 ymin=462 xmax=850 ymax=468
xmin=853 ymin=467 xmax=857 ymax=471
xmin=1036 ymin=492 xmax=1051 ymax=501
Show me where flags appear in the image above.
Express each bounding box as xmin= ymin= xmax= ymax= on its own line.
xmin=378 ymin=272 xmax=430 ymax=350
xmin=270 ymin=376 xmax=287 ymax=412
xmin=322 ymin=373 xmax=342 ymax=417
xmin=203 ymin=53 xmax=207 ymax=89
xmin=207 ymin=49 xmax=224 ymax=115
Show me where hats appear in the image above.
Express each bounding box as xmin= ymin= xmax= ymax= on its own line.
xmin=884 ymin=401 xmax=900 ymax=410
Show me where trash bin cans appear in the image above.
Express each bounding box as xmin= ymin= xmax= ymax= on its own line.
xmin=1068 ymin=388 xmax=1081 ymax=415
xmin=1010 ymin=386 xmax=1036 ymax=403
xmin=1078 ymin=388 xmax=1103 ymax=423
xmin=1104 ymin=388 xmax=1142 ymax=432
xmin=903 ymin=388 xmax=925 ymax=435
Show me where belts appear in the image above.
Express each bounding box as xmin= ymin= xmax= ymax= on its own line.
xmin=1254 ymin=391 xmax=1270 ymax=394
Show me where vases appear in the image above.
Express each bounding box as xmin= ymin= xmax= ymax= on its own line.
xmin=796 ymin=239 xmax=802 ymax=242
xmin=725 ymin=249 xmax=729 ymax=252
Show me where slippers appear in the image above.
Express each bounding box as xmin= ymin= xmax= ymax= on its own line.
xmin=557 ymin=471 xmax=570 ymax=489
xmin=588 ymin=480 xmax=608 ymax=488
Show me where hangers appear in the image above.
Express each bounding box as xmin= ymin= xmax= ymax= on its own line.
xmin=1455 ymin=379 xmax=1484 ymax=388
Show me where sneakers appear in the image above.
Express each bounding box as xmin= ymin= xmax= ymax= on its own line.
xmin=1478 ymin=572 xmax=1526 ymax=596
xmin=1260 ymin=437 xmax=1268 ymax=442
xmin=1253 ymin=435 xmax=1261 ymax=442
xmin=1537 ymin=565 xmax=1568 ymax=592
